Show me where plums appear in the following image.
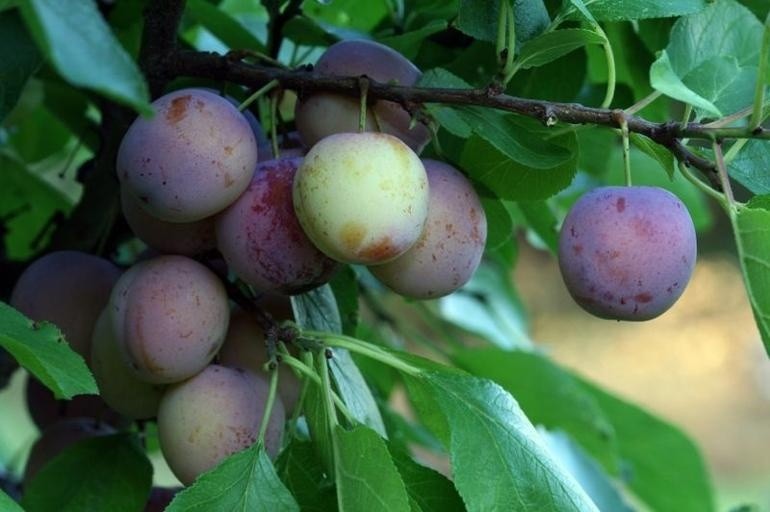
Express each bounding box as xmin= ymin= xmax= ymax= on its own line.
xmin=558 ymin=124 xmax=697 ymax=321
xmin=8 ymin=41 xmax=489 ymax=488
